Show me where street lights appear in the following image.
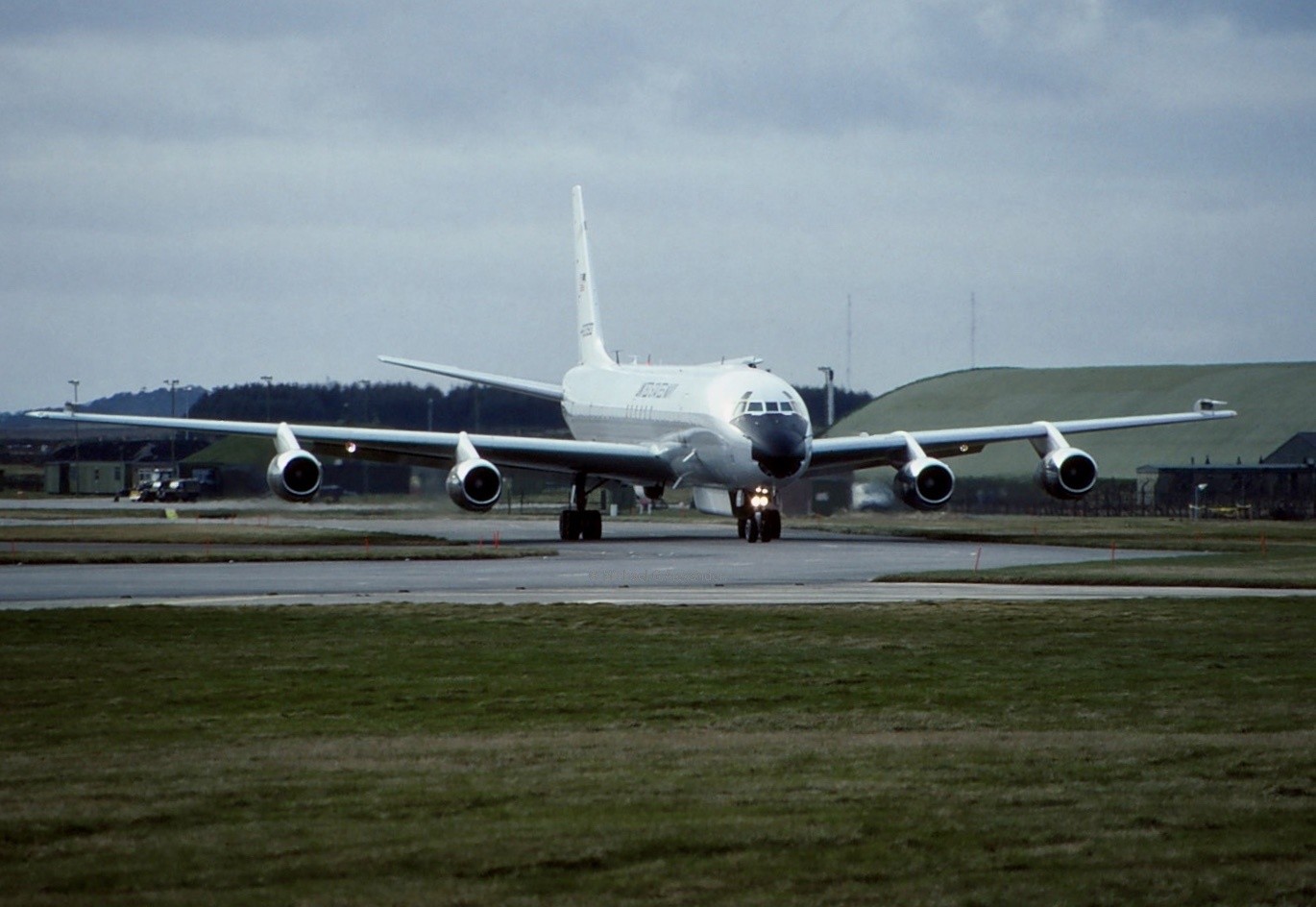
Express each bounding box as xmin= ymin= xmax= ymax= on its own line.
xmin=162 ymin=379 xmax=180 ymax=476
xmin=259 ymin=375 xmax=274 ymax=421
xmin=67 ymin=379 xmax=80 ymax=496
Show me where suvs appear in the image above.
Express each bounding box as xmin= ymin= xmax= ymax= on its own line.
xmin=135 ymin=477 xmax=201 ymax=504
xmin=191 ymin=466 xmax=224 ymax=497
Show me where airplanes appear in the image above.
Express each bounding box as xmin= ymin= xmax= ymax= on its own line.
xmin=23 ymin=185 xmax=1239 ymax=548
